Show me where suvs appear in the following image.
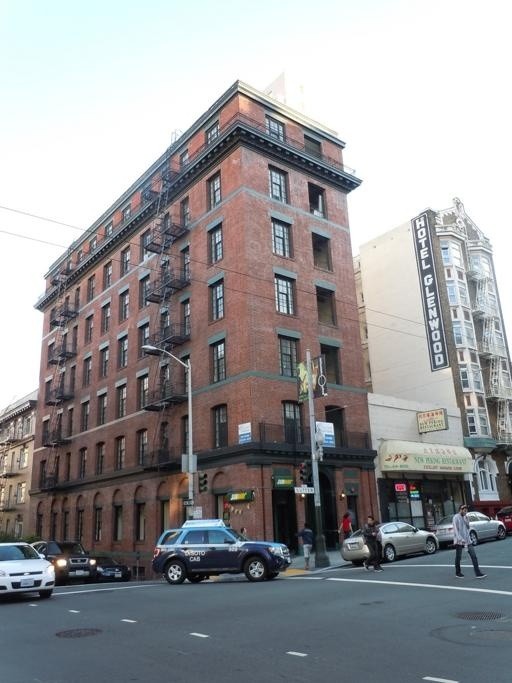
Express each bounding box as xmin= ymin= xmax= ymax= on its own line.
xmin=28 ymin=539 xmax=97 ymax=585
xmin=152 ymin=519 xmax=292 ymax=583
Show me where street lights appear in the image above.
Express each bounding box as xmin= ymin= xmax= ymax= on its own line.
xmin=140 ymin=344 xmax=197 ymax=518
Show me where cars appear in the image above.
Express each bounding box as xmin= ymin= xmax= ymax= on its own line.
xmin=432 ymin=511 xmax=507 ymax=550
xmin=495 ymin=503 xmax=512 ymax=534
xmin=0 ymin=542 xmax=56 ymax=600
xmin=340 ymin=521 xmax=439 ymax=566
xmin=89 ymin=555 xmax=132 ymax=581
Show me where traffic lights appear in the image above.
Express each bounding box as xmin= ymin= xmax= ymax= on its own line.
xmin=199 ymin=473 xmax=208 ymax=493
xmin=299 ymin=460 xmax=312 ymax=489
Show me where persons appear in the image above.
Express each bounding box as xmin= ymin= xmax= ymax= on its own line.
xmin=338 ymin=511 xmax=353 ymax=540
xmin=363 ymin=515 xmax=384 ymax=572
xmin=238 ymin=527 xmax=248 ymax=541
xmin=295 ymin=523 xmax=313 ymax=570
xmin=375 ymin=520 xmax=383 ymax=567
xmin=452 ymin=504 xmax=487 ymax=579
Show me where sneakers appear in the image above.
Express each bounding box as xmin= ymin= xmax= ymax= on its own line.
xmin=363 ymin=562 xmax=369 ymax=570
xmin=456 ymin=573 xmax=464 ymax=578
xmin=475 ymin=574 xmax=487 ymax=579
xmin=374 ymin=568 xmax=384 ymax=572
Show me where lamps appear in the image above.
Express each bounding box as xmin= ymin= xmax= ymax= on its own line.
xmin=340 ymin=490 xmax=346 ymax=501
